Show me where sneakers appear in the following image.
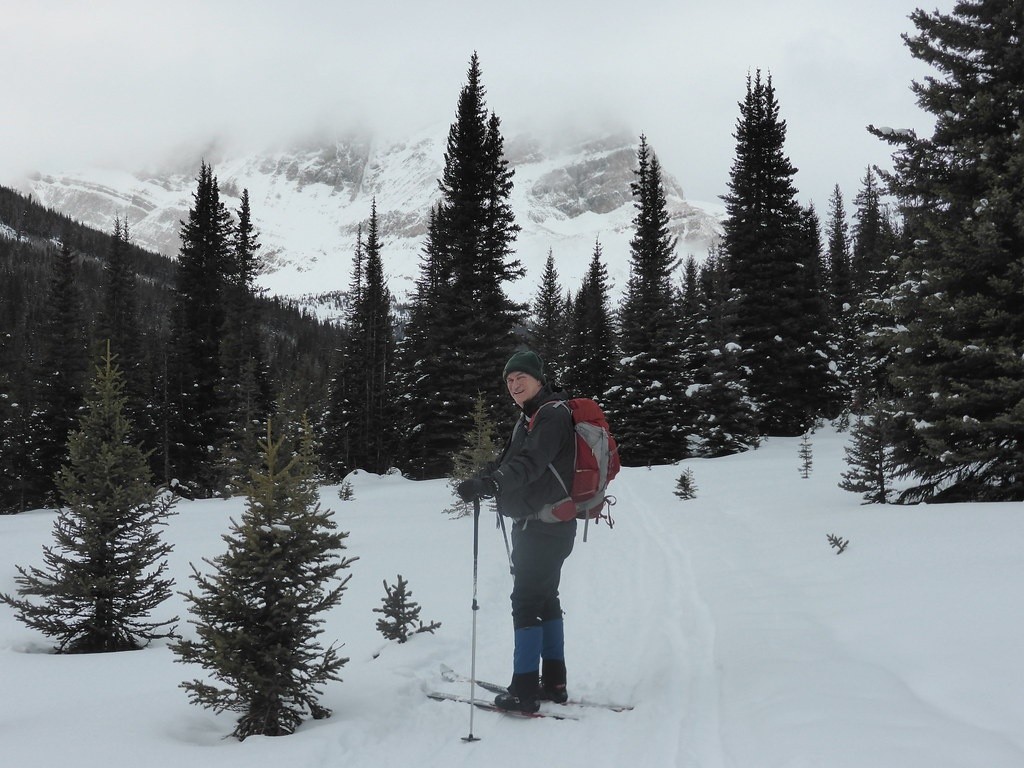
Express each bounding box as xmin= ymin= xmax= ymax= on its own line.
xmin=495 ymin=692 xmax=540 ymax=713
xmin=538 ymin=676 xmax=568 ymax=704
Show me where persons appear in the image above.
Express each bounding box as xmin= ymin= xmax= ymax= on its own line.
xmin=456 ymin=351 xmax=579 ymax=712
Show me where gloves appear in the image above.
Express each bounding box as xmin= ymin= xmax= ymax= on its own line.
xmin=473 ymin=466 xmax=490 ymax=478
xmin=458 ymin=478 xmax=488 ymax=503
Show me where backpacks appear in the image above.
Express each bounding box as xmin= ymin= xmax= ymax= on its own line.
xmin=512 ymin=398 xmax=620 ymax=520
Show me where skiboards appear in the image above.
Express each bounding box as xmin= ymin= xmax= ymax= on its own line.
xmin=421 ymin=664 xmax=636 ymax=721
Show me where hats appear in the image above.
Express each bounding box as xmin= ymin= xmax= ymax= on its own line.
xmin=503 ymin=351 xmax=547 ymax=386
